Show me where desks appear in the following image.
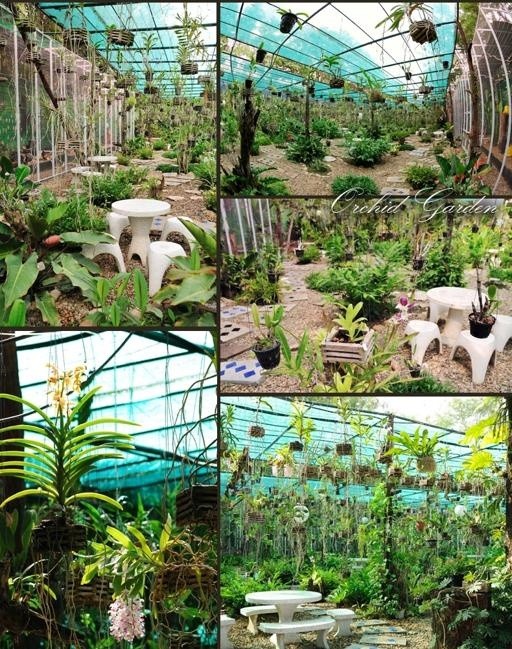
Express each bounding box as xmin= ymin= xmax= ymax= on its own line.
xmin=245 ymin=590 xmax=322 ymax=646
xmin=113 ymin=199 xmax=171 ymax=263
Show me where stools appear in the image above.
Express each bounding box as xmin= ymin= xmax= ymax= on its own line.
xmin=146 ymin=240 xmax=186 ymax=295
xmin=220 ymin=615 xmax=238 ymax=647
xmin=82 ymin=235 xmax=127 ymax=281
xmin=161 ymin=217 xmax=198 ymax=255
xmin=328 ymin=607 xmax=353 ymax=640
xmin=108 ymin=213 xmax=131 ymax=248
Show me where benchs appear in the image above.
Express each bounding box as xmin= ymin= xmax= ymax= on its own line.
xmin=258 ymin=618 xmax=337 ymax=649
xmin=239 ymin=604 xmax=306 ymax=632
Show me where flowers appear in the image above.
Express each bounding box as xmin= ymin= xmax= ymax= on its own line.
xmin=85 ymin=518 xmax=212 ymax=643
xmin=3 ymin=364 xmax=143 ymax=518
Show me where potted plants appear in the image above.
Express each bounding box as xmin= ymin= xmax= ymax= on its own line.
xmin=238 ymin=2 xmax=470 ymax=102
xmin=223 ymin=219 xmax=512 ymax=383
xmin=223 ymin=398 xmax=511 ymax=585
xmin=13 ymin=3 xmax=216 ymax=148
